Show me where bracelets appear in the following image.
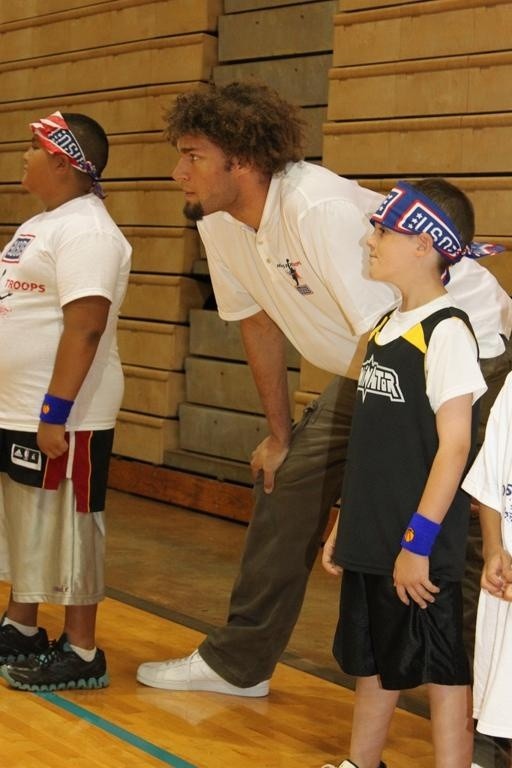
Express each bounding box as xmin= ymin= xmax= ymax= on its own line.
xmin=39 ymin=392 xmax=73 ymax=425
xmin=400 ymin=512 xmax=439 ymax=556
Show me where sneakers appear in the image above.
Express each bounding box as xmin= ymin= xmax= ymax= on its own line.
xmin=0 ymin=614 xmax=108 ymax=691
xmin=137 ymin=650 xmax=270 ymax=696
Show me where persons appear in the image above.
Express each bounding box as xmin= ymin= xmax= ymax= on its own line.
xmin=459 ymin=371 xmax=511 ymax=768
xmin=320 ymin=176 xmax=492 ymax=766
xmin=134 ymin=80 xmax=512 ymax=700
xmin=0 ymin=113 xmax=132 ymax=693
